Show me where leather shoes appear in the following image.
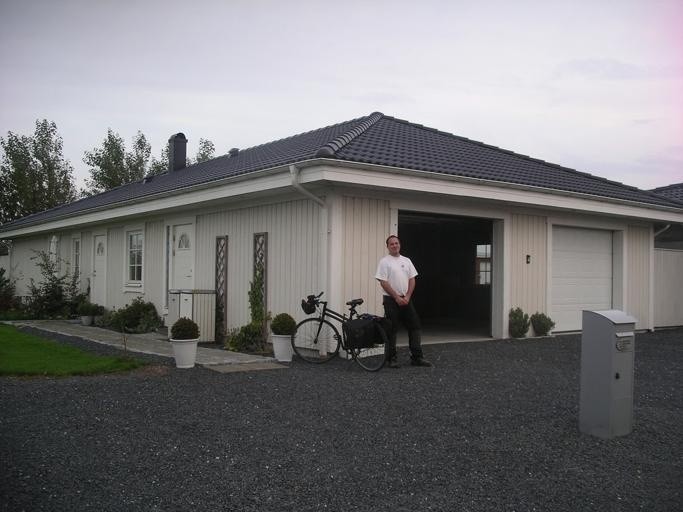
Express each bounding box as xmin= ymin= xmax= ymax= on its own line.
xmin=385 ymin=360 xmax=400 ymax=368
xmin=411 ymin=357 xmax=432 ymax=367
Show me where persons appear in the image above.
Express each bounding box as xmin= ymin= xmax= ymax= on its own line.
xmin=375 ymin=235 xmax=433 ymax=368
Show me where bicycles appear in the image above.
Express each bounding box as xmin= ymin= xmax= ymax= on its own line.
xmin=291 ymin=292 xmax=389 ymax=371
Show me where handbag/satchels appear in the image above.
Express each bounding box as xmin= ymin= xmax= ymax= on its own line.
xmin=342 ymin=318 xmax=376 ymax=349
xmin=363 ymin=312 xmax=392 ymax=345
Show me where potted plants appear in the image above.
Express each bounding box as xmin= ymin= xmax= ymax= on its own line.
xmin=168 ymin=316 xmax=200 ymax=370
xmin=269 ymin=313 xmax=298 ymax=363
xmin=76 ymin=300 xmax=104 ymax=326
